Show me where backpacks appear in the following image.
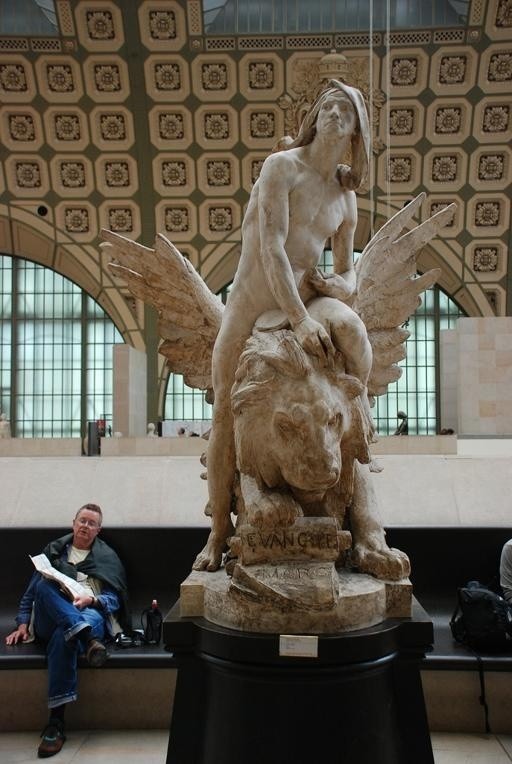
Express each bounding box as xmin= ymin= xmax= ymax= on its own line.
xmin=448 ymin=585 xmax=512 ymax=658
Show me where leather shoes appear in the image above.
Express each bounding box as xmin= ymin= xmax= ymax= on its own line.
xmin=37 ymin=727 xmax=66 ymax=759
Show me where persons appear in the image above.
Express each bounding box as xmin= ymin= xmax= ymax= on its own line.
xmin=189 ymin=78 xmax=386 ymax=573
xmin=394 ymin=410 xmax=408 ymax=436
xmin=146 ymin=421 xmax=159 ymax=438
xmin=497 ymin=537 xmax=512 ymax=639
xmin=4 ymin=503 xmax=127 ymax=758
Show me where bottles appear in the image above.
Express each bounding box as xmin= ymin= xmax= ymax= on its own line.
xmin=143 ymin=600 xmax=163 ymax=645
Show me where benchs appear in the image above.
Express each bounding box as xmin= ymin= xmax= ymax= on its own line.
xmin=0 ymin=525 xmax=512 ymax=734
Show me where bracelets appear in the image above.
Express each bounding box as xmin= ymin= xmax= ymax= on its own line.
xmin=90 ymin=595 xmax=95 ymax=606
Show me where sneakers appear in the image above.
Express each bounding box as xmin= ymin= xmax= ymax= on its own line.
xmin=86 ymin=640 xmax=110 ymax=669
